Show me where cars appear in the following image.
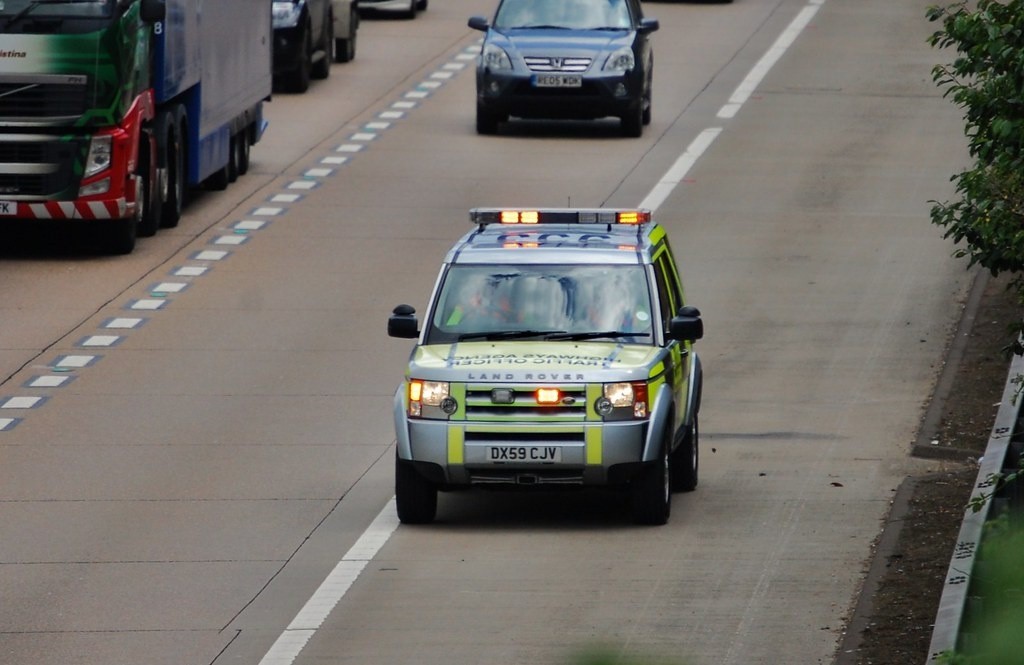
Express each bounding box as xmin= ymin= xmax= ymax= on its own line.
xmin=467 ymin=0 xmax=662 ymax=136
xmin=354 ymin=0 xmax=428 ymax=21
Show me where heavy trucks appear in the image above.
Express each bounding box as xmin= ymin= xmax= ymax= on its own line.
xmin=273 ymin=0 xmax=361 ymax=93
xmin=0 ymin=0 xmax=275 ymax=253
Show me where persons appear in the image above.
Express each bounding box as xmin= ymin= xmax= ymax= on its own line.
xmin=574 ymin=274 xmax=652 ymax=335
xmin=447 ymin=274 xmax=523 ymax=327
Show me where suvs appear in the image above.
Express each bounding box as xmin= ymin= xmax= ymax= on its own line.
xmin=386 ymin=205 xmax=704 ymax=525
